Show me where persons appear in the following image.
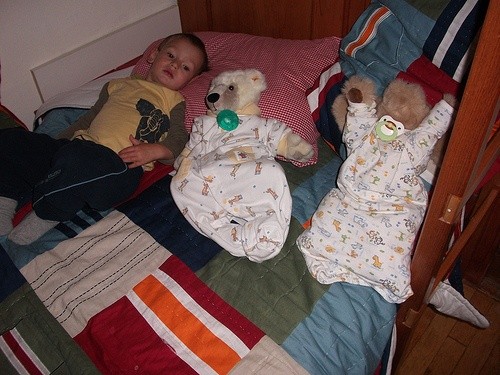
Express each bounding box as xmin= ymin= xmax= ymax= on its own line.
xmin=0 ymin=33 xmax=210 ymax=246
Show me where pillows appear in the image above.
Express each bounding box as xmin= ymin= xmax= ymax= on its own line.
xmin=129 ymin=30 xmax=342 ymax=167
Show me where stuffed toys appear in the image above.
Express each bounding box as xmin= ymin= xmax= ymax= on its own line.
xmin=170 ymin=71 xmax=314 ymax=263
xmin=295 ymin=70 xmax=456 ymax=304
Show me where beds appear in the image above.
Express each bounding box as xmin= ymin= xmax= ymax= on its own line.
xmin=0 ymin=45 xmax=468 ymax=375
